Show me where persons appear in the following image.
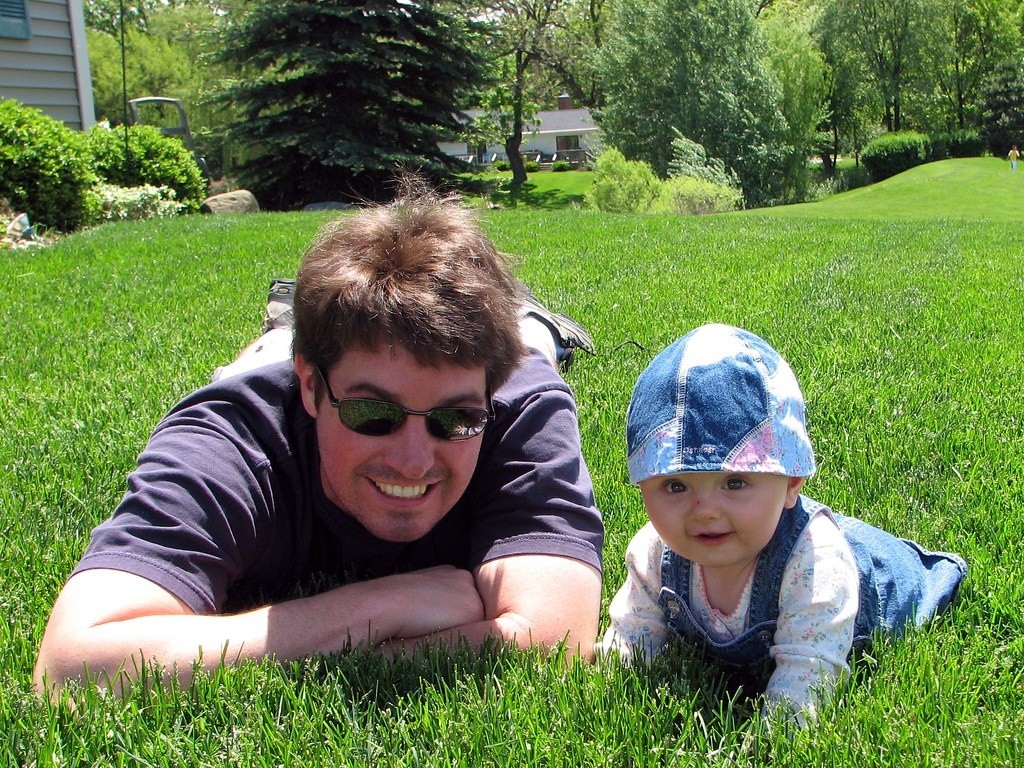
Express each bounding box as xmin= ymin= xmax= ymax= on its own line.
xmin=30 ymin=190 xmax=604 ymax=719
xmin=594 ymin=323 xmax=968 ymax=741
xmin=1007 ymin=145 xmax=1020 ymax=170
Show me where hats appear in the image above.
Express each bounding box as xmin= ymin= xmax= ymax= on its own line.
xmin=626 ymin=324 xmax=816 ymax=486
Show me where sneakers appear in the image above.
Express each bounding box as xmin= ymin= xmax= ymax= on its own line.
xmin=515 ymin=280 xmax=644 ymax=374
xmin=268 ymin=279 xmax=296 ymax=306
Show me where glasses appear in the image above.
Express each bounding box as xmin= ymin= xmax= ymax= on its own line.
xmin=315 ymin=365 xmax=495 ymax=443
